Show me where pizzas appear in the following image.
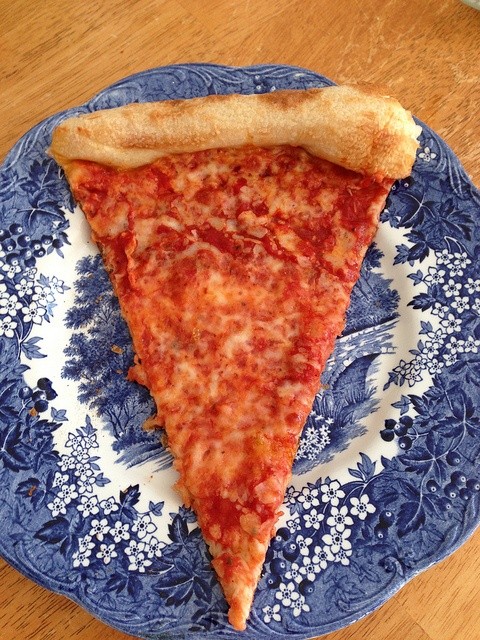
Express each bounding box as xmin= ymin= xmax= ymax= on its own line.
xmin=51 ymin=84 xmax=422 ymax=636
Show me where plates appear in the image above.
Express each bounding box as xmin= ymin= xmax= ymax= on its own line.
xmin=0 ymin=65 xmax=480 ymax=640
